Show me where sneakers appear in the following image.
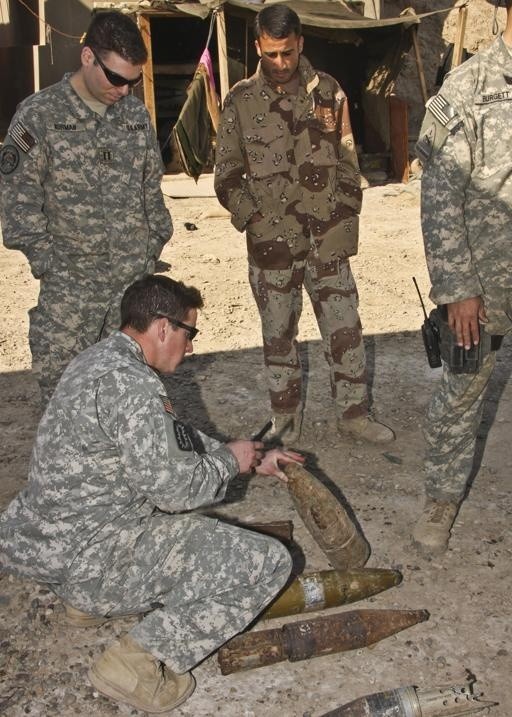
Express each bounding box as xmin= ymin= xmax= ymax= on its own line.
xmin=345 ymin=415 xmax=395 ymax=443
xmin=275 ymin=413 xmax=302 ymax=446
xmin=85 ymin=634 xmax=196 ymax=714
xmin=62 ymin=600 xmax=109 ymax=628
xmin=411 ymin=497 xmax=460 ymax=555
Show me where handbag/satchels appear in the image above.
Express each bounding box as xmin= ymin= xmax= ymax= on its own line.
xmin=430 ymin=306 xmax=485 ymax=377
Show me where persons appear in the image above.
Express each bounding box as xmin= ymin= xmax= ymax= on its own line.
xmin=216 ymin=3 xmax=397 ymax=443
xmin=0 ymin=273 xmax=304 ymax=713
xmin=403 ymin=0 xmax=512 ymax=554
xmin=0 ymin=10 xmax=173 ymax=416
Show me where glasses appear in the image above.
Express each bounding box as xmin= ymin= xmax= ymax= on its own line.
xmin=91 ymin=45 xmax=143 ymax=90
xmin=152 ymin=311 xmax=199 ymax=340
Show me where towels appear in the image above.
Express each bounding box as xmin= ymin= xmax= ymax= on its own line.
xmin=173 ymin=49 xmax=220 ymax=184
